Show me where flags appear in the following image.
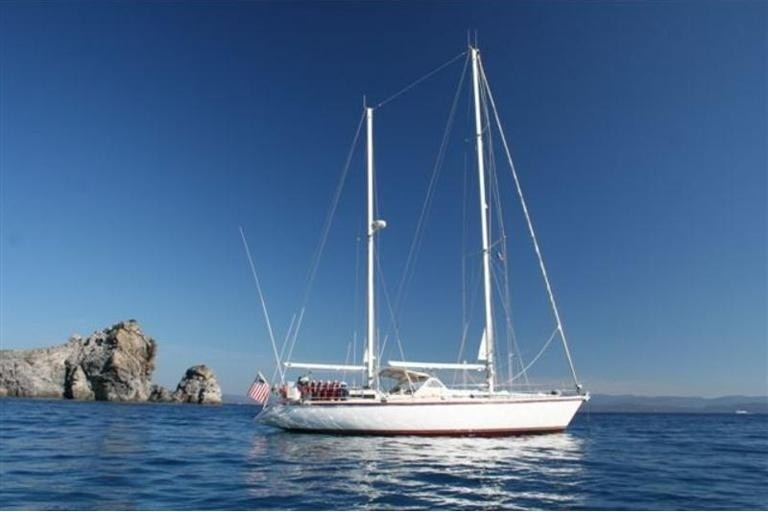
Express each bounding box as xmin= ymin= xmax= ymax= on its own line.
xmin=245 ymin=373 xmax=269 ymax=404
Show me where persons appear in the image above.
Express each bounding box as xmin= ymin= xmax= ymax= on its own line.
xmin=295 ymin=377 xmax=347 ymax=401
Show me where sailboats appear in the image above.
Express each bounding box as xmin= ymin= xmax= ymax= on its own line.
xmin=239 ymin=40 xmax=590 ymax=439
